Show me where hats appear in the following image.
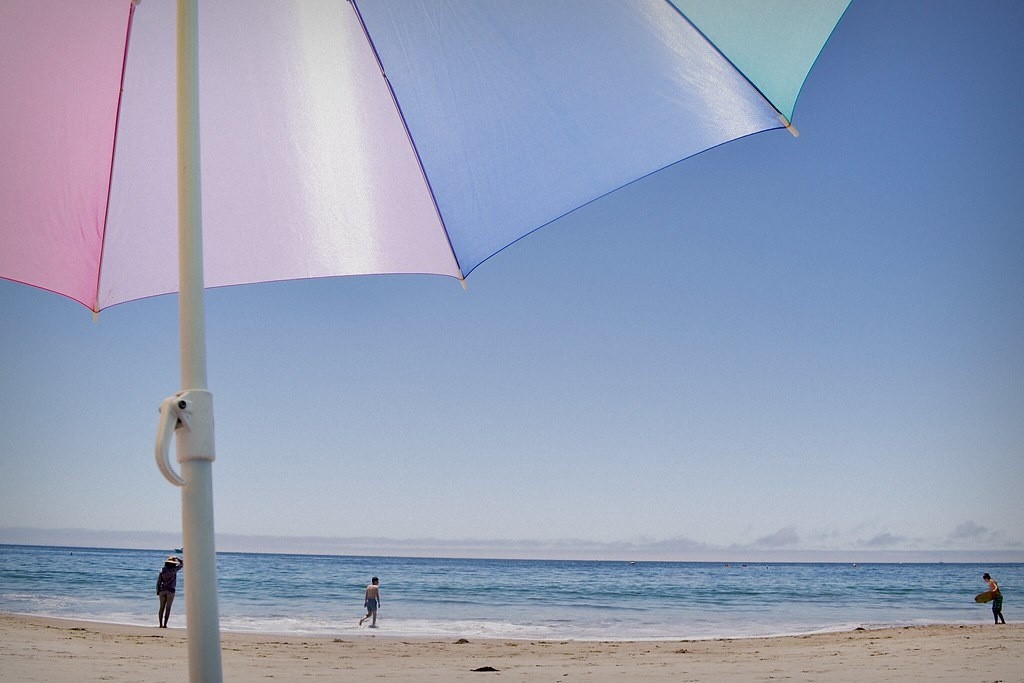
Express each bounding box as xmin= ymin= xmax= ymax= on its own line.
xmin=165 ymin=556 xmax=179 ymax=564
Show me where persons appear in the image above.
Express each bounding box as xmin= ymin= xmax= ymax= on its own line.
xmin=983 ymin=573 xmax=1005 ymax=624
xmin=359 ymin=577 xmax=380 ymax=628
xmin=156 ymin=556 xmax=183 ymax=628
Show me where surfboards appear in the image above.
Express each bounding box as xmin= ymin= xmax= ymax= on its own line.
xmin=975 ymin=590 xmax=1000 ymax=603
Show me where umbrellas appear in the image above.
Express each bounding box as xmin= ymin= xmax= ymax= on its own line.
xmin=0 ymin=0 xmax=851 ymax=683
